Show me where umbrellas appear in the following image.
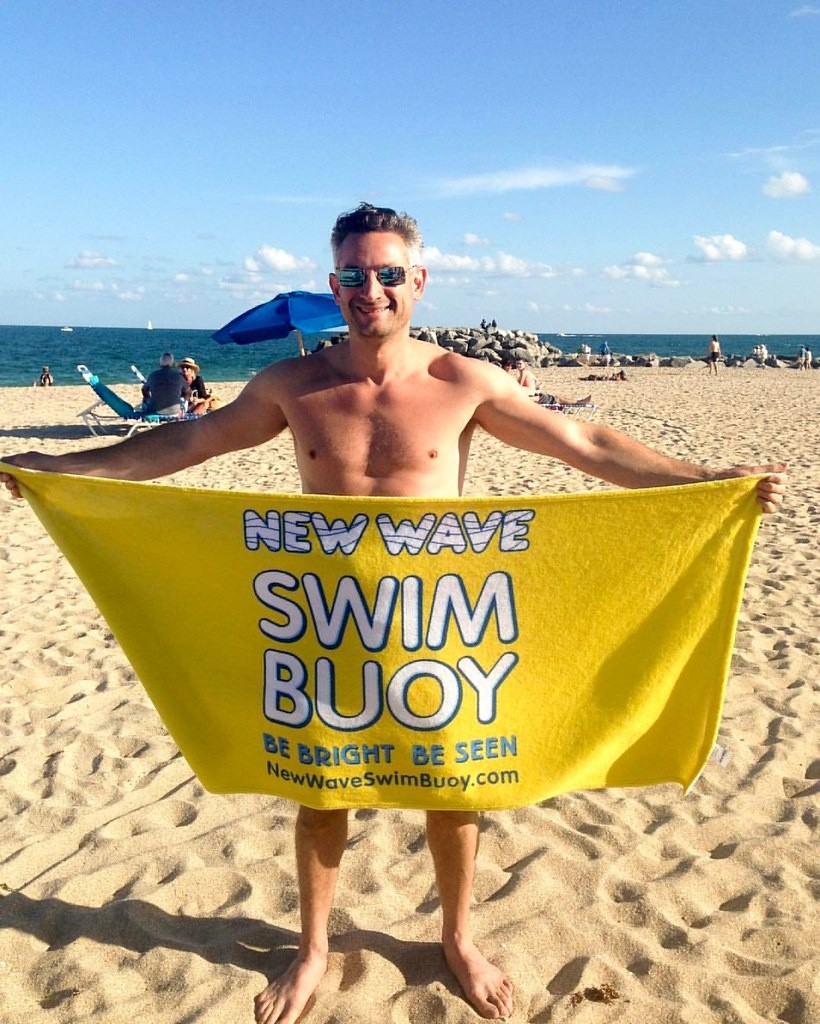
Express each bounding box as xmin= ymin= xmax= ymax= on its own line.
xmin=212 ymin=291 xmax=350 ymax=357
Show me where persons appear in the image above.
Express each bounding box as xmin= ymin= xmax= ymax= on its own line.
xmin=797 ymin=345 xmax=812 ymax=370
xmin=752 ymin=344 xmax=768 ymax=368
xmin=479 ymin=318 xmax=497 ymax=333
xmin=141 ymin=353 xmax=217 ymax=420
xmin=0 ymin=201 xmax=788 ymax=1023
xmin=39 ymin=364 xmax=53 ymax=385
xmin=578 ymin=339 xmax=610 ymax=356
xmin=709 ymin=335 xmax=722 ymax=375
xmin=502 ymin=357 xmax=592 ymax=405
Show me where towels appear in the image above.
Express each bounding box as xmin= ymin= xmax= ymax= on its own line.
xmin=0 ymin=460 xmax=776 ymax=814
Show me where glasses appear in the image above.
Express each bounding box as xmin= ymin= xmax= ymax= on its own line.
xmin=338 ymin=265 xmax=417 ymax=287
xmin=181 ymin=365 xmax=191 ymax=369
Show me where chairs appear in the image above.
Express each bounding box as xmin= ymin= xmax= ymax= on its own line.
xmin=131 ymin=365 xmax=188 ymax=413
xmin=75 ymin=364 xmax=207 ymax=436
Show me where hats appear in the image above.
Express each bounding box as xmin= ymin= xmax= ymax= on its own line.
xmin=176 ymin=358 xmax=200 ymax=370
